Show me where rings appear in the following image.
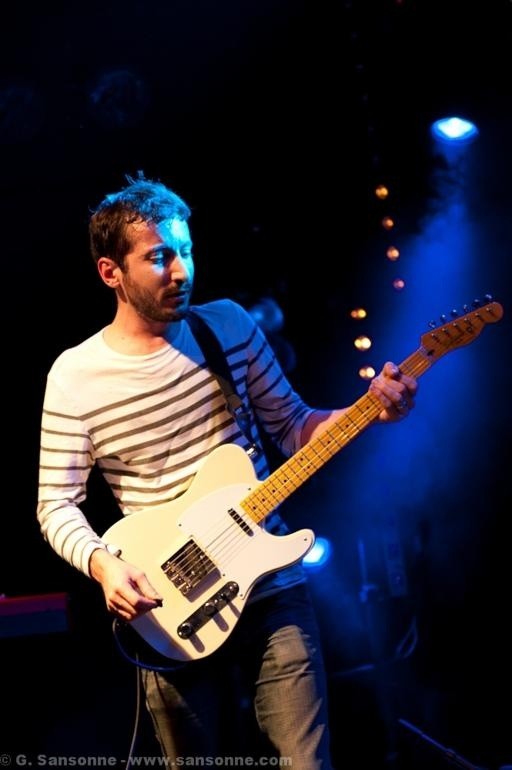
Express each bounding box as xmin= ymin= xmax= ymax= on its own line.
xmin=398 ymin=400 xmax=408 ymax=410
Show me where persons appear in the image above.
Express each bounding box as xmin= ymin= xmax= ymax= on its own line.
xmin=36 ymin=170 xmax=417 ymax=769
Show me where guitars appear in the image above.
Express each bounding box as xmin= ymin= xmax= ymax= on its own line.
xmin=99 ymin=294 xmax=504 ymax=664
